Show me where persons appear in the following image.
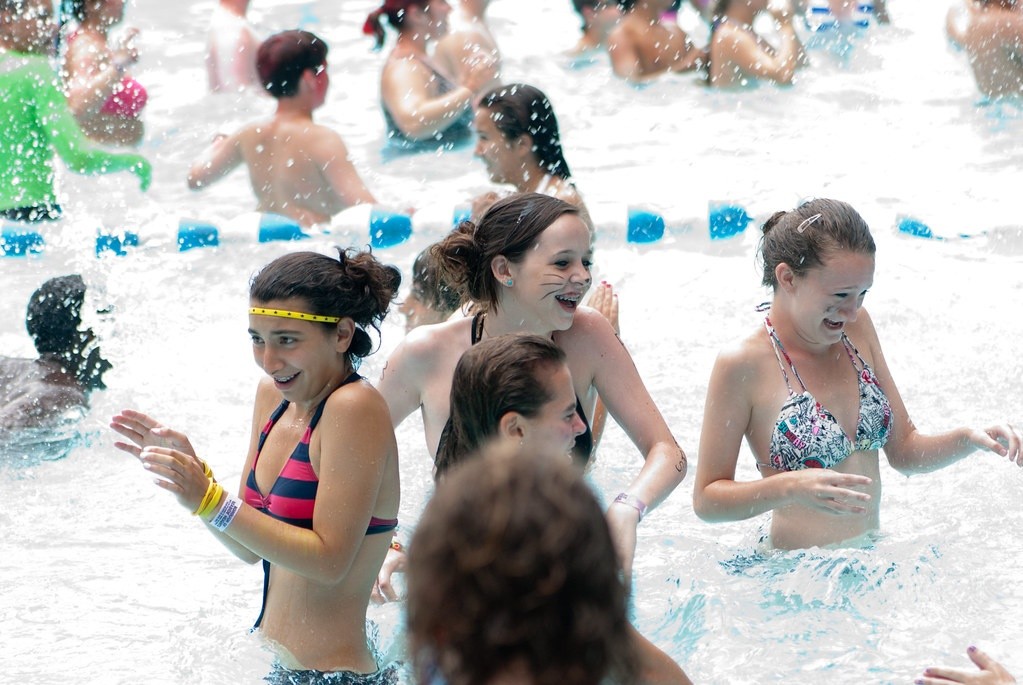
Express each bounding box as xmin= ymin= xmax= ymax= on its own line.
xmin=362 ymin=0 xmax=476 ymax=155
xmin=110 ymin=244 xmax=400 ymax=673
xmin=204 ymin=0 xmax=264 ymax=91
xmin=432 ymin=0 xmax=502 ymax=86
xmin=0 ymin=0 xmax=153 ymax=224
xmin=61 ymin=0 xmax=148 ymax=146
xmin=693 ymin=198 xmax=1023 ymax=551
xmin=469 ymin=83 xmax=596 ymax=233
xmin=914 ymin=645 xmax=1017 ymax=685
xmin=405 ymin=453 xmax=694 ymax=685
xmin=187 ymin=29 xmax=379 ymax=228
xmin=397 ymin=220 xmax=620 ymax=446
xmin=432 ymin=333 xmax=587 ymax=482
xmin=0 ymin=274 xmax=113 ymax=428
xmin=562 ymin=0 xmax=1023 ymax=95
xmin=370 ymin=193 xmax=688 ymax=606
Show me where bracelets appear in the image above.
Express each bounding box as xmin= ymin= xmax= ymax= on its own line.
xmin=390 ymin=541 xmax=406 ymax=555
xmin=191 ymin=477 xmax=243 ymax=532
xmin=202 ymin=461 xmax=213 ymax=477
xmin=614 ymin=493 xmax=647 ymax=522
